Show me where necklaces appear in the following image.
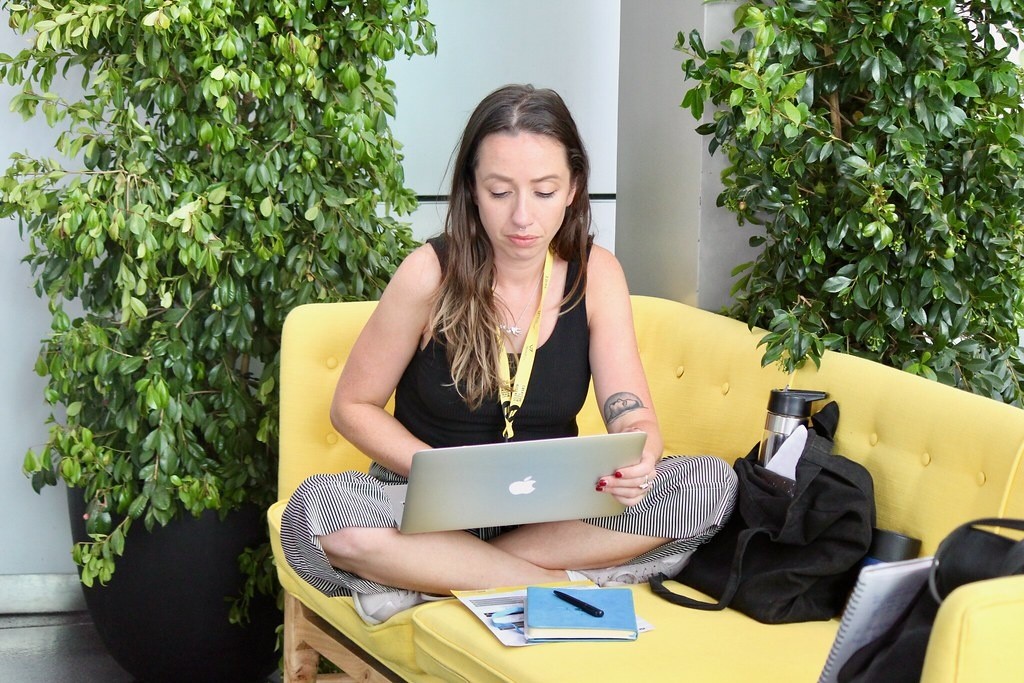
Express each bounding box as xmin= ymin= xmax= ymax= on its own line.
xmin=499 ymin=273 xmax=543 ymax=337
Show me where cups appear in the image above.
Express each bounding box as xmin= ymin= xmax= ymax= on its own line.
xmin=862 ymin=527 xmax=925 ymax=570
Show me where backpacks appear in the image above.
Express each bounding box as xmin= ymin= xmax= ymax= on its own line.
xmin=648 ymin=400 xmax=876 ymax=625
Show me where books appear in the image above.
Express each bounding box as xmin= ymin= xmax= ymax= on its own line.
xmin=815 ymin=555 xmax=939 ymax=683
xmin=524 ymin=587 xmax=639 ymax=643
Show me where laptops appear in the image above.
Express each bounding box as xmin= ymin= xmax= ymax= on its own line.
xmin=383 ymin=432 xmax=650 ymax=535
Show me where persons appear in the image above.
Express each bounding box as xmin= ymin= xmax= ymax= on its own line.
xmin=279 ymin=83 xmax=739 ymax=597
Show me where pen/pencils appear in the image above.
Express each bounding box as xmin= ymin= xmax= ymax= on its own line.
xmin=552 ymin=589 xmax=605 ymax=618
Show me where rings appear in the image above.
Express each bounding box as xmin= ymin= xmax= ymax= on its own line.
xmin=639 ymin=475 xmax=649 ymax=490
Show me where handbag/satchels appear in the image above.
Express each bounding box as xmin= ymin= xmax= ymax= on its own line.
xmin=837 ymin=517 xmax=1024 ymax=683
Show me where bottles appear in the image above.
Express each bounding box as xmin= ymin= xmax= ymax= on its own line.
xmin=757 ymin=385 xmax=829 ymax=471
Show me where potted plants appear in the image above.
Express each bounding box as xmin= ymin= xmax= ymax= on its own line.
xmin=0 ymin=0 xmax=440 ymax=683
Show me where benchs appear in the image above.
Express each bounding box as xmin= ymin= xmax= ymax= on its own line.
xmin=266 ymin=296 xmax=1024 ymax=683
